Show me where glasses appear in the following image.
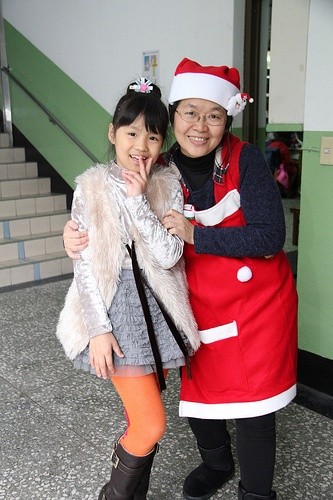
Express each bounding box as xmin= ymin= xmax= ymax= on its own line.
xmin=175 ymin=109 xmax=227 ymax=126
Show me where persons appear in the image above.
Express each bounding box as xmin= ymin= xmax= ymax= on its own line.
xmin=62 ymin=58 xmax=298 ymax=500
xmin=56 ymin=80 xmax=203 ymax=500
xmin=264 ymin=130 xmax=301 ymax=197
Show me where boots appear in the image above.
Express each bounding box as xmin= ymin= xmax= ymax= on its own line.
xmin=98 ymin=434 xmax=159 ymax=500
xmin=237 ymin=481 xmax=277 ymax=500
xmin=183 ymin=432 xmax=235 ymax=500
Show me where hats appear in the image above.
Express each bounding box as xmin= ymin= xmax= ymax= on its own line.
xmin=168 ymin=58 xmax=254 ymax=117
